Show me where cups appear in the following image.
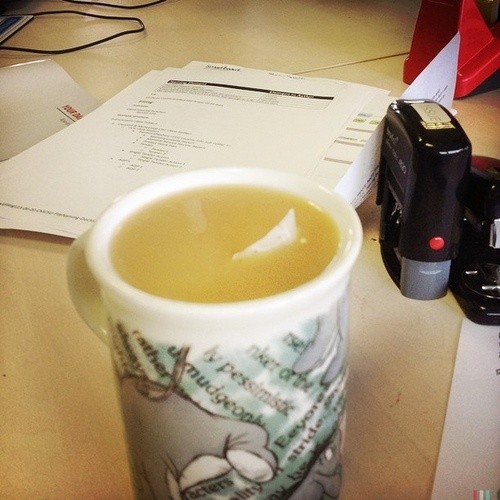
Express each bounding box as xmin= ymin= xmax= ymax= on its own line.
xmin=66 ymin=169 xmax=363 ymax=500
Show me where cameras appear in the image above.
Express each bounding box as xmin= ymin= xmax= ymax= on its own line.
xmin=376 ymin=98 xmax=500 ymax=326
